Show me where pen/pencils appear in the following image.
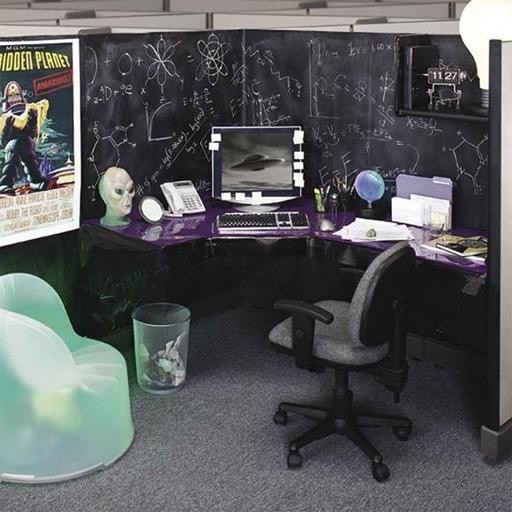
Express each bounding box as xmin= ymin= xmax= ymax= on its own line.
xmin=314 ymin=177 xmax=355 ymax=213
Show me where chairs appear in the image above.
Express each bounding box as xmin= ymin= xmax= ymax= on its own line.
xmin=269 ymin=242 xmax=416 ymax=481
xmin=0 ymin=273 xmax=134 ymax=484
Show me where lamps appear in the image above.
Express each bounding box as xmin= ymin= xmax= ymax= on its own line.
xmin=459 ymin=0 xmax=512 ymax=110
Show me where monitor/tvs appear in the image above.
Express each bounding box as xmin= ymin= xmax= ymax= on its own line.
xmin=211 ymin=125 xmax=302 ymax=212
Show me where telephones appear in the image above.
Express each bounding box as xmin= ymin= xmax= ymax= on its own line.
xmin=160 ymin=180 xmax=207 ymax=215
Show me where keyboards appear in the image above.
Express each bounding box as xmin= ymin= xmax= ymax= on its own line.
xmin=216 ymin=211 xmax=310 ymax=231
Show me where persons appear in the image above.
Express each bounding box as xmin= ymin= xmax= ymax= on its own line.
xmin=98 ymin=167 xmax=136 ymax=228
xmin=0 ymin=80 xmax=50 ymax=195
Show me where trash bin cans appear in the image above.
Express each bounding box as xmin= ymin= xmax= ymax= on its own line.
xmin=131 ymin=302 xmax=191 ymax=394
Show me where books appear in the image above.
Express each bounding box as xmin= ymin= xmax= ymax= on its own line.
xmin=420 ymin=234 xmax=489 ymax=262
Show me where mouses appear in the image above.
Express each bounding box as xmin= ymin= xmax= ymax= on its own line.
xmin=318 ymin=218 xmax=336 ymax=232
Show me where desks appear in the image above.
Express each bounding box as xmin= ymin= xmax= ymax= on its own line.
xmin=78 ymin=196 xmax=489 ymax=334
